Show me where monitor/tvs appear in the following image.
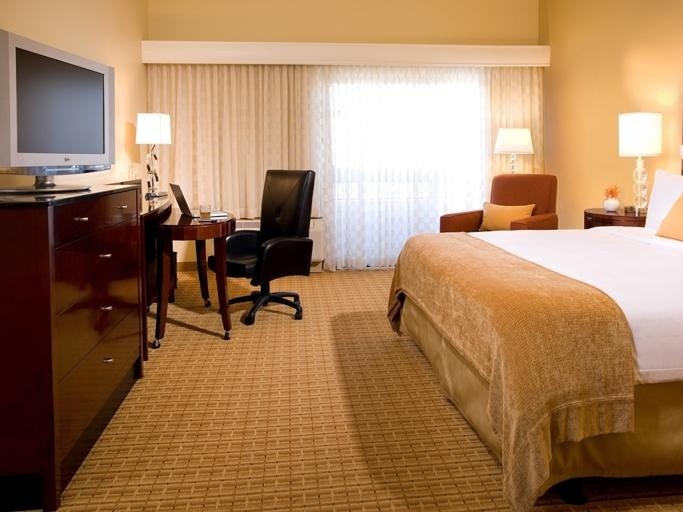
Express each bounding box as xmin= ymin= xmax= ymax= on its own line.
xmin=0 ymin=29 xmax=115 ymax=195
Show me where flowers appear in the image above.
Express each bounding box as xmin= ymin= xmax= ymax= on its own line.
xmin=605 ymin=184 xmax=620 ymax=198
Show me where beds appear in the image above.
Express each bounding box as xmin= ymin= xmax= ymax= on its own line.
xmin=386 ymin=171 xmax=683 ymax=511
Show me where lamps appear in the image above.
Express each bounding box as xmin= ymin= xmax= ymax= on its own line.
xmin=618 ymin=112 xmax=663 ymax=214
xmin=135 ymin=113 xmax=172 ymax=198
xmin=492 ymin=128 xmax=536 ymax=174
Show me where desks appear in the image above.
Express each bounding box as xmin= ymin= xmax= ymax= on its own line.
xmin=151 ymin=209 xmax=236 ymax=349
xmin=140 ymin=190 xmax=172 ymax=361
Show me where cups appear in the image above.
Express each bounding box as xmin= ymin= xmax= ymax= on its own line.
xmin=200 ymin=204 xmax=211 ymax=219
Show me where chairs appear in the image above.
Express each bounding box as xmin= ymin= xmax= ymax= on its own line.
xmin=440 ymin=173 xmax=558 ymax=232
xmin=208 ymin=169 xmax=315 ymax=326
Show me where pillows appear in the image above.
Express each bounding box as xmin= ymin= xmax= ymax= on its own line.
xmin=479 ymin=201 xmax=536 ymax=231
xmin=643 ymin=169 xmax=683 ymax=234
xmin=654 ymin=192 xmax=683 ymax=241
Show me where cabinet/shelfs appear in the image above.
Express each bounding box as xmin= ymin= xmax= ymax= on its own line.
xmin=1 ymin=185 xmax=140 ymax=511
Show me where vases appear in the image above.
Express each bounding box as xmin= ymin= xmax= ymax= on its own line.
xmin=603 ymin=197 xmax=619 ymax=212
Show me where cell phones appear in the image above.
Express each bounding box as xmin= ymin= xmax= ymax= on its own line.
xmin=198 ymin=219 xmax=218 ymax=223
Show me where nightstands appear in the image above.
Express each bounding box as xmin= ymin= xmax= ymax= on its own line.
xmin=584 ymin=207 xmax=647 ymax=229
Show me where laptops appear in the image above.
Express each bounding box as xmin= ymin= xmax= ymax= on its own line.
xmin=169 ymin=183 xmax=228 ymax=218
